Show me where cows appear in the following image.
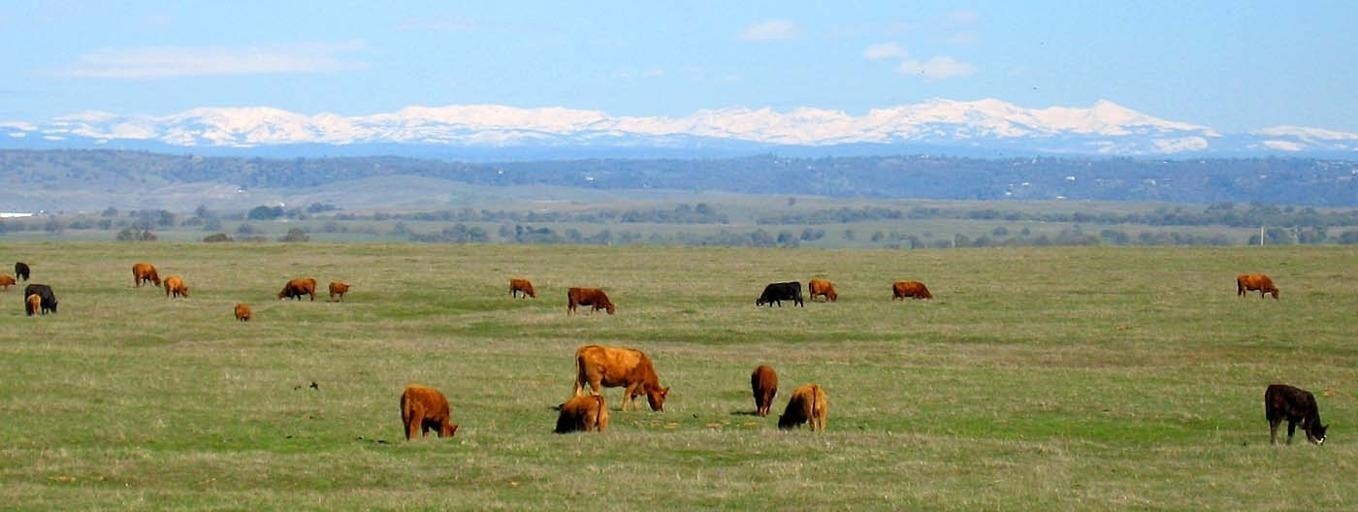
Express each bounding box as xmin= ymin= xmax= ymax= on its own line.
xmin=1264 ymin=384 xmax=1329 ymax=447
xmin=277 ymin=276 xmax=351 ymax=302
xmin=132 ymin=263 xmax=189 ymax=300
xmin=233 ymin=302 xmax=249 ymax=322
xmin=1236 ymin=273 xmax=1279 ymax=300
xmin=0 ymin=260 xmax=56 ymax=319
xmin=891 ymin=280 xmax=933 ymax=302
xmin=754 ymin=278 xmax=838 ymax=307
xmin=508 ymin=278 xmax=536 ymax=299
xmin=552 ymin=344 xmax=670 ymax=435
xmin=751 ymin=364 xmax=828 ymax=431
xmin=400 ymin=384 xmax=459 ymax=441
xmin=568 ymin=287 xmax=616 ymax=315
